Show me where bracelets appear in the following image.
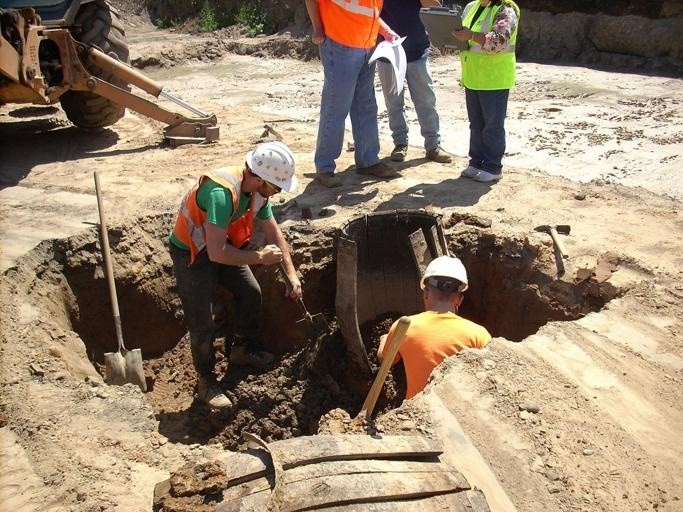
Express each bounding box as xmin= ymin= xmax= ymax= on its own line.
xmin=470 ymin=31 xmax=474 ymax=40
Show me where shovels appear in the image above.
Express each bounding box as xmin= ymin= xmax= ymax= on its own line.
xmin=277 ymin=262 xmax=331 ymax=343
xmin=534 ymin=225 xmax=570 ymax=258
xmin=94 ymin=171 xmax=147 ymax=392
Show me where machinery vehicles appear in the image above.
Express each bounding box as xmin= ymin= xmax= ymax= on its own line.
xmin=0 ymin=0 xmax=219 ymax=149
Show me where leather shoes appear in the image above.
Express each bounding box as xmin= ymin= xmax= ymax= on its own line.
xmin=390 ymin=145 xmax=408 ymax=162
xmin=357 ymin=162 xmax=400 ymax=179
xmin=426 ymin=148 xmax=453 ymax=163
xmin=315 ymin=171 xmax=343 ymax=188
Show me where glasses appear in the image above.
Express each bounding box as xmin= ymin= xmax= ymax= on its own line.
xmin=264 ymin=181 xmax=282 ymax=195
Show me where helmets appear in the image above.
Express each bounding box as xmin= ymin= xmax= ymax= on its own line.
xmin=246 ymin=141 xmax=295 ymax=193
xmin=419 ymin=256 xmax=470 ymax=292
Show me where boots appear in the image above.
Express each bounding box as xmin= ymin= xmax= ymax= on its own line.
xmin=230 ymin=334 xmax=277 ymax=370
xmin=196 ymin=372 xmax=233 ymax=408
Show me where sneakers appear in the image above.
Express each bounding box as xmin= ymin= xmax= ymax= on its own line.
xmin=473 ymin=171 xmax=504 ymax=184
xmin=461 ymin=166 xmax=479 ymax=178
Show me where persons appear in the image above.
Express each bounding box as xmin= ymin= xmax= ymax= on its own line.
xmin=448 ymin=0 xmax=520 ymax=182
xmin=375 ymin=0 xmax=452 ymax=164
xmin=304 ymin=0 xmax=403 ymax=189
xmin=169 ymin=140 xmax=301 ymax=411
xmin=377 ymin=255 xmax=493 ymax=402
